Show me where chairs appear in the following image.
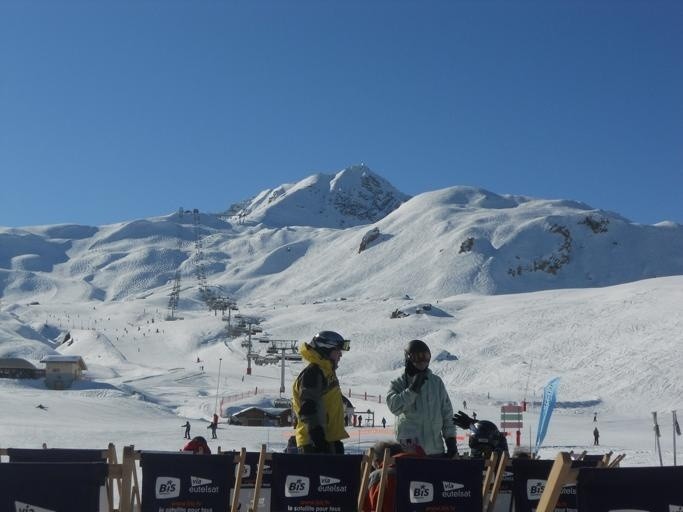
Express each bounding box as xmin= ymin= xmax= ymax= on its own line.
xmin=0 ymin=444 xmax=683 ymax=512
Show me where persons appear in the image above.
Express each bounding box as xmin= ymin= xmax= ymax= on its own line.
xmin=451 ymin=410 xmax=509 ymax=459
xmin=385 ymin=340 xmax=458 ymax=456
xmin=205 ymin=421 xmax=217 ymax=439
xmin=180 ymin=421 xmax=191 ymax=440
xmin=592 ymin=428 xmax=599 ymax=446
xmin=291 ymin=330 xmax=350 ymax=455
xmin=361 ymin=441 xmax=425 ymax=512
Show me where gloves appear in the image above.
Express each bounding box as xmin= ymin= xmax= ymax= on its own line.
xmin=408 ymin=372 xmax=429 ymax=394
xmin=310 ymin=438 xmax=330 ymax=453
xmin=450 ymin=410 xmax=476 ymax=431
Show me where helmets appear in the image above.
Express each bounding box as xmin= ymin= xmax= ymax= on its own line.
xmin=306 ymin=330 xmax=351 ymax=371
xmin=468 ymin=419 xmax=501 ymax=453
xmin=402 ymin=339 xmax=431 ymax=378
